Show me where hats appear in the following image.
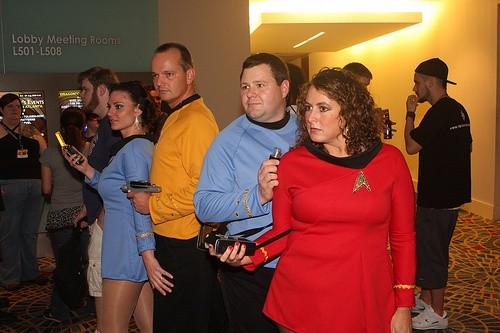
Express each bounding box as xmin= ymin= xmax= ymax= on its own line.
xmin=414 ymin=57 xmax=458 ymax=85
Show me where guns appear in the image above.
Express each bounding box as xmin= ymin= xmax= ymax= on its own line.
xmin=120 ymin=180 xmax=161 ymax=193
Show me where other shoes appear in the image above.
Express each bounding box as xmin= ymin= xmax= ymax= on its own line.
xmin=31 ymin=275 xmax=49 ymax=285
xmin=43 ymin=310 xmax=62 ymax=323
xmin=2 ymin=280 xmax=19 ymax=290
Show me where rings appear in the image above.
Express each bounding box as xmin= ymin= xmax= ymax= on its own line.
xmin=159 ymin=277 xmax=163 ymax=280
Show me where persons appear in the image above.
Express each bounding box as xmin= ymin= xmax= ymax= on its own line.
xmin=0 ymin=93 xmax=52 ymax=291
xmin=61 ymin=79 xmax=173 ymax=333
xmin=405 ymin=58 xmax=472 ymax=330
xmin=81 ymin=105 xmax=103 ymax=143
xmin=127 ymin=42 xmax=220 ymax=332
xmin=286 ymin=62 xmax=307 ymax=107
xmin=192 ymin=54 xmax=308 ymax=333
xmin=342 ymin=62 xmax=372 ymax=90
xmin=209 ymin=66 xmax=417 ymax=333
xmin=76 ymin=66 xmax=118 ymax=333
xmin=39 ymin=106 xmax=96 ymax=324
xmin=144 ymin=85 xmax=169 ymax=143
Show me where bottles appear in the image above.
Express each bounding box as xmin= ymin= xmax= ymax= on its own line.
xmin=383 ymin=109 xmax=392 ymax=139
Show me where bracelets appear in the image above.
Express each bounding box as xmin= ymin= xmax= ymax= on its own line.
xmin=406 ymin=111 xmax=415 ymax=121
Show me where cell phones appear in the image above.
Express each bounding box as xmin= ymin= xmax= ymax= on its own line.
xmin=63 ymin=146 xmax=84 ymax=164
xmin=214 ymin=239 xmax=256 ymax=257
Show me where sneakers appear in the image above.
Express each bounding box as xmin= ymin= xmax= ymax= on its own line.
xmin=411 ymin=293 xmax=426 ymax=313
xmin=411 ymin=305 xmax=448 ymax=330
